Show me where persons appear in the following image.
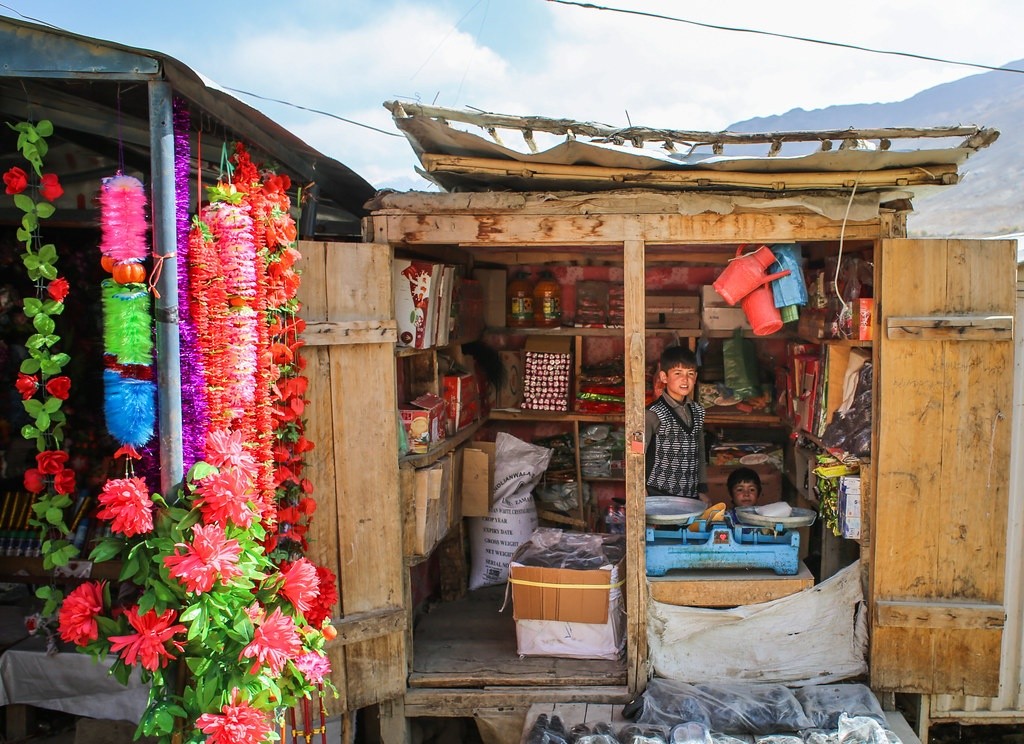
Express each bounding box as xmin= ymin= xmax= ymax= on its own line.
xmin=727 ymin=467 xmax=762 ymax=514
xmin=644 ymin=347 xmax=713 ymax=530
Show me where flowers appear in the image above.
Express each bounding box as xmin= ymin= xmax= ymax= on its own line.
xmin=4 ymin=122 xmax=337 ymax=744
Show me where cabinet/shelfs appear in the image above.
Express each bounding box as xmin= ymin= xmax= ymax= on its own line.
xmin=397 ymin=324 xmax=872 ymax=579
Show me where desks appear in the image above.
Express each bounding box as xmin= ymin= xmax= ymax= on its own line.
xmin=649 ymin=560 xmax=815 ymax=607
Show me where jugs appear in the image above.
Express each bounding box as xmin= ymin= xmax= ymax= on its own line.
xmin=740 ymin=270 xmax=784 ymax=337
xmin=711 ymin=242 xmax=791 ymax=307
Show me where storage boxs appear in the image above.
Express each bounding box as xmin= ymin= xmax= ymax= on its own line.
xmin=499 ymin=542 xmax=626 ymax=661
xmin=644 ymin=284 xmax=753 ymax=331
xmin=390 ymin=259 xmax=574 ymax=554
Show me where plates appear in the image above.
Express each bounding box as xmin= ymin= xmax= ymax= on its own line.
xmin=739 ymin=453 xmax=770 ymax=465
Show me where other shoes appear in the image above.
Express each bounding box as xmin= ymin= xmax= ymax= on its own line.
xmin=524 ymin=682 xmax=902 ymax=744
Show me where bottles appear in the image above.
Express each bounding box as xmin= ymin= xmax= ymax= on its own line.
xmin=505 ymin=271 xmax=535 ymax=328
xmin=606 ymin=502 xmax=626 ymax=535
xmin=529 ymin=270 xmax=563 ymax=329
